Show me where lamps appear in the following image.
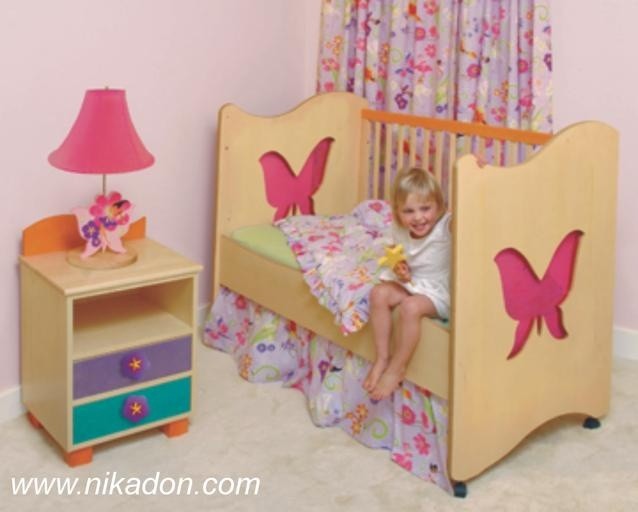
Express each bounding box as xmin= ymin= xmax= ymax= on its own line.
xmin=47 ymin=88 xmax=158 ymax=271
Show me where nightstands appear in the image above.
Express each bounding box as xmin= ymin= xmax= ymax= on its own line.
xmin=18 ymin=214 xmax=205 ymax=468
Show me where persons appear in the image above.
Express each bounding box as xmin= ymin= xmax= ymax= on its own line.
xmin=362 ymin=167 xmax=452 ymax=405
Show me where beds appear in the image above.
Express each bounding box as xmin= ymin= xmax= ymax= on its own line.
xmin=211 ymin=91 xmax=618 ymax=498
